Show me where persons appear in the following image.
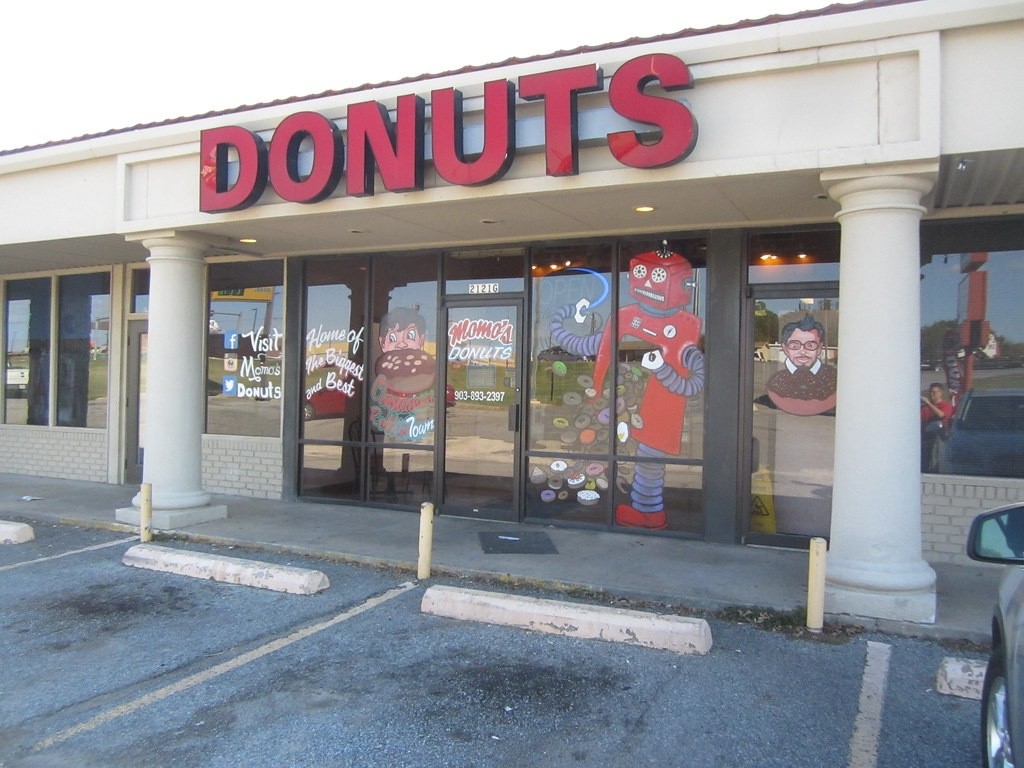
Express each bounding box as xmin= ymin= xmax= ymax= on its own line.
xmin=920 ymin=382 xmax=957 ymax=474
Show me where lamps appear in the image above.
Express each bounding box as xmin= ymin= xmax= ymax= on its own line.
xmin=759 ymin=245 xmax=784 ymax=262
xmin=548 ymin=258 xmax=560 ymax=270
xmin=561 ymin=255 xmax=572 ymax=267
xmin=796 ymin=242 xmax=810 ymax=260
xmin=531 ymin=261 xmax=540 ymax=272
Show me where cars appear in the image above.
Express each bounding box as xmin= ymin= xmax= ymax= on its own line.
xmin=931 ymin=388 xmax=1023 ymax=479
xmin=966 ymin=502 xmax=1024 ymax=768
xmin=537 ymin=345 xmax=593 ymax=362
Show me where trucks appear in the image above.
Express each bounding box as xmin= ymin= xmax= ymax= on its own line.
xmin=6 ymin=352 xmax=29 ymax=399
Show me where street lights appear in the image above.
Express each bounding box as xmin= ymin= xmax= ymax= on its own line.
xmin=251 ymin=308 xmax=258 ymax=332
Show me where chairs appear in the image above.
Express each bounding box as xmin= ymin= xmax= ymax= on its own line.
xmin=348 ymin=420 xmax=399 ymax=502
